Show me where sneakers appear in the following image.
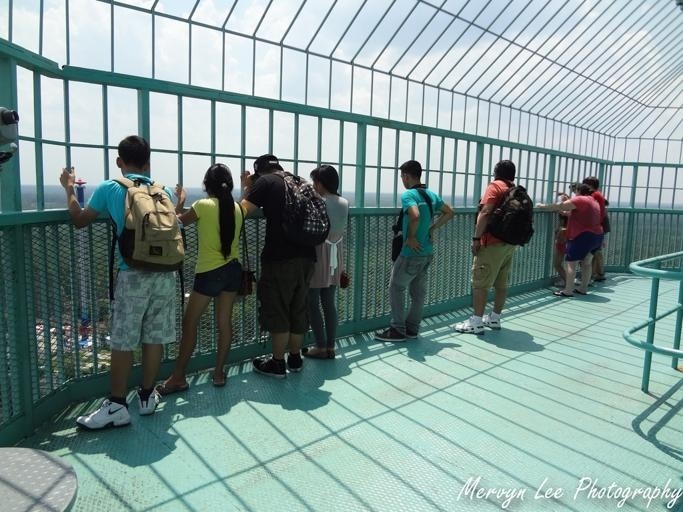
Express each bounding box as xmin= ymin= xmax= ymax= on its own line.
xmin=76 ymin=392 xmax=131 ymax=429
xmin=376 ymin=328 xmax=418 ymax=342
xmin=455 ymin=311 xmax=500 ymax=335
xmin=137 ymin=386 xmax=159 ymax=416
xmin=287 ymin=353 xmax=302 ymax=372
xmin=253 ymin=356 xmax=287 ymax=377
xmin=302 ymin=345 xmax=335 ymax=359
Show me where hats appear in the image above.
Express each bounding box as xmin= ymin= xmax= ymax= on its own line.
xmin=250 ymin=154 xmax=283 ymax=179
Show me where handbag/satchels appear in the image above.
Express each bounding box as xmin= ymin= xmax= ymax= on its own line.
xmin=239 ymin=266 xmax=256 ymax=295
xmin=392 ymin=231 xmax=403 ymax=260
xmin=339 ymin=269 xmax=350 ymax=288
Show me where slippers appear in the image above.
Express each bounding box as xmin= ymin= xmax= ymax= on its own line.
xmin=156 ymin=378 xmax=189 ymax=394
xmin=553 ymin=271 xmax=607 ymax=296
xmin=213 ymin=372 xmax=225 ymax=385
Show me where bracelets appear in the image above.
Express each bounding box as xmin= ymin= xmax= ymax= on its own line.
xmin=472 ymin=237 xmax=480 ymax=242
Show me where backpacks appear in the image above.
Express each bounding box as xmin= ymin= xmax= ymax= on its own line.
xmin=115 ymin=176 xmax=185 ymax=273
xmin=274 ymin=169 xmax=330 ymax=245
xmin=493 ymin=177 xmax=534 ymax=244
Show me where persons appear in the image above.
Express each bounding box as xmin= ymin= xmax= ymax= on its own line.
xmin=455 ymin=158 xmax=517 ymax=334
xmin=155 ymin=163 xmax=247 ymax=397
xmin=239 ymin=155 xmax=309 ymax=380
xmin=535 ymin=175 xmax=613 ymax=297
xmin=373 ymin=160 xmax=455 ymax=343
xmin=301 ymin=165 xmax=349 ymax=359
xmin=60 ymin=133 xmax=179 ymax=432
